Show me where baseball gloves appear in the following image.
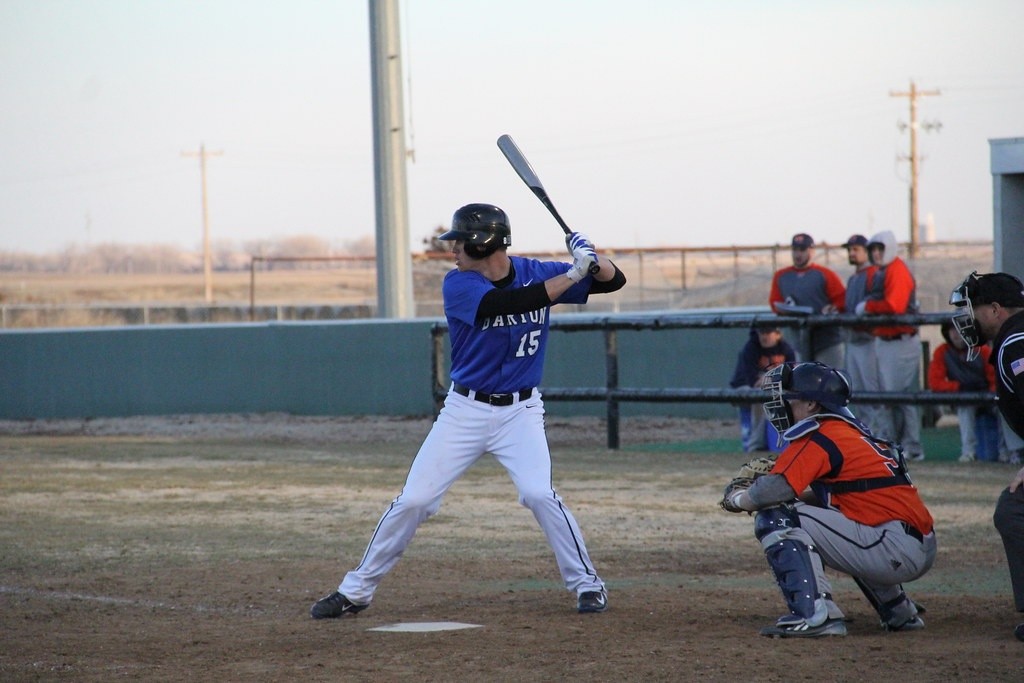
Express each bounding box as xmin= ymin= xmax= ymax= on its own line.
xmin=721 ymin=456 xmax=775 ymax=513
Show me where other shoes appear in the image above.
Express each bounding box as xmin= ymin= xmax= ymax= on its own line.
xmin=958 ymin=452 xmax=975 ymax=462
xmin=1009 ymin=451 xmax=1020 ymax=464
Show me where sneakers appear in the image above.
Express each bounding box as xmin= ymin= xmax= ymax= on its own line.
xmin=880 ymin=615 xmax=926 ymax=630
xmin=760 ymin=621 xmax=846 ymax=639
xmin=574 ymin=591 xmax=606 ymax=612
xmin=308 ymin=591 xmax=370 ymax=618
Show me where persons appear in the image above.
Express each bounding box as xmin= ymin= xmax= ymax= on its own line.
xmin=729 ymin=233 xmax=845 ymax=451
xmin=928 ymin=312 xmax=1009 ymax=463
xmin=311 ymin=203 xmax=626 ymax=618
xmin=720 ymin=361 xmax=936 ymax=636
xmin=949 ymin=271 xmax=1024 ymax=647
xmin=839 ymin=231 xmax=925 ymax=462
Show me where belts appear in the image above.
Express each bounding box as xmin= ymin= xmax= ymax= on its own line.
xmin=901 ymin=524 xmax=936 ymax=543
xmin=453 ymin=382 xmax=532 ymax=406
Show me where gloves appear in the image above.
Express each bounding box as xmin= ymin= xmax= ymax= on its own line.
xmin=564 ymin=231 xmax=599 ymax=283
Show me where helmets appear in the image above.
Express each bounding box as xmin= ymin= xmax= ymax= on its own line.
xmin=438 ymin=203 xmax=511 ymax=257
xmin=784 ymin=362 xmax=856 ymax=419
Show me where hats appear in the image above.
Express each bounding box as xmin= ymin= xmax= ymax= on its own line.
xmin=790 ymin=233 xmax=813 ymax=249
xmin=842 ymin=235 xmax=867 ymax=249
xmin=966 ymin=271 xmax=1023 ymax=307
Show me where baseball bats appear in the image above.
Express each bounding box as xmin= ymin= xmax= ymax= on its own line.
xmin=496 ymin=133 xmax=601 ymax=275
